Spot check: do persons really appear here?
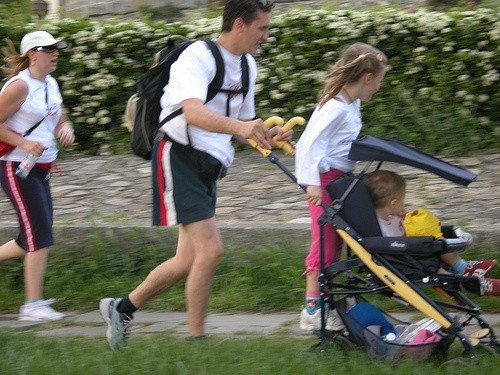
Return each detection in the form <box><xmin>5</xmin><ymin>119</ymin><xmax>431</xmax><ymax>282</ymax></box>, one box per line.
<box><xmin>295</xmin><ymin>43</ymin><xmax>388</xmax><ymax>332</ymax></box>
<box><xmin>99</xmin><ymin>0</ymin><xmax>293</xmax><ymax>352</ymax></box>
<box><xmin>362</xmin><ymin>170</ymin><xmax>500</xmax><ymax>296</ymax></box>
<box><xmin>0</xmin><ymin>30</ymin><xmax>77</xmax><ymax>322</ymax></box>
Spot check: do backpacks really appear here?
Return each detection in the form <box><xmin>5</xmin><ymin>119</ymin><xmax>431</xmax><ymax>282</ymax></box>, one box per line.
<box><xmin>127</xmin><ymin>34</ymin><xmax>251</xmax><ymax>158</ymax></box>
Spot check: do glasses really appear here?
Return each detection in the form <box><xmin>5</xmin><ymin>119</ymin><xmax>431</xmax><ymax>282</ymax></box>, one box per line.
<box><xmin>31</xmin><ymin>45</ymin><xmax>58</xmax><ymax>53</ymax></box>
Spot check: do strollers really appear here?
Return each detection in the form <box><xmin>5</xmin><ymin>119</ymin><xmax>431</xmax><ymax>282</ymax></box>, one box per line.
<box><xmin>246</xmin><ymin>115</ymin><xmax>500</xmax><ymax>367</ymax></box>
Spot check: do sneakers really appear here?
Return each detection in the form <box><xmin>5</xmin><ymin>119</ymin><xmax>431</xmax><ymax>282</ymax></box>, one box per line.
<box><xmin>17</xmin><ymin>298</ymin><xmax>65</xmax><ymax>322</ymax></box>
<box><xmin>476</xmin><ymin>277</ymin><xmax>500</xmax><ymax>297</ymax></box>
<box><xmin>462</xmin><ymin>258</ymin><xmax>497</xmax><ymax>278</ymax></box>
<box><xmin>299</xmin><ymin>307</ymin><xmax>345</xmax><ymax>331</ymax></box>
<box><xmin>99</xmin><ymin>298</ymin><xmax>136</xmax><ymax>351</ymax></box>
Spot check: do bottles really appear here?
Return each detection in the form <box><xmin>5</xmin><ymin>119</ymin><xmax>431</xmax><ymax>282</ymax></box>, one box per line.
<box><xmin>368</xmin><ymin>333</ymin><xmax>396</xmax><ymax>362</ymax></box>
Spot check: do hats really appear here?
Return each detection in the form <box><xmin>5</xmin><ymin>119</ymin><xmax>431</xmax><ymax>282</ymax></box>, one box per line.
<box><xmin>19</xmin><ymin>31</ymin><xmax>68</xmax><ymax>57</ymax></box>
